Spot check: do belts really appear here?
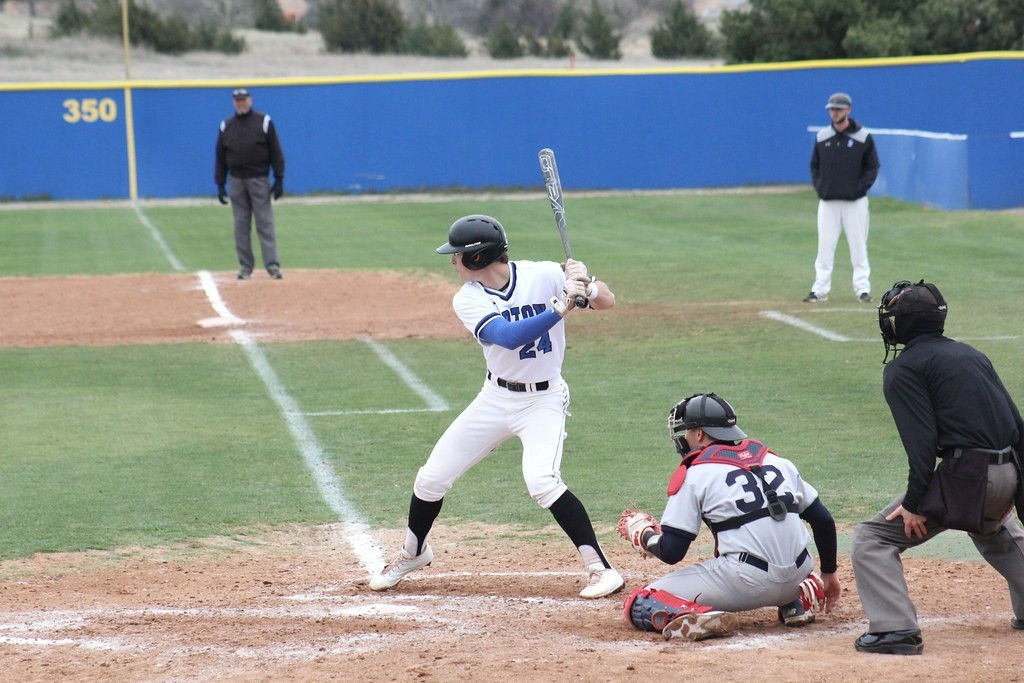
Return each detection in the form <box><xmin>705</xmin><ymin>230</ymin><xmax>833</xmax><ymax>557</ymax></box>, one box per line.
<box><xmin>948</xmin><ymin>447</ymin><xmax>1013</xmax><ymax>465</ymax></box>
<box><xmin>739</xmin><ymin>548</ymin><xmax>808</xmax><ymax>572</ymax></box>
<box><xmin>487</xmin><ymin>371</ymin><xmax>549</xmax><ymax>392</ymax></box>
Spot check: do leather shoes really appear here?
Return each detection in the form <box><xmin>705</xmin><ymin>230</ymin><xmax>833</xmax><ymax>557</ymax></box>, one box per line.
<box><xmin>854</xmin><ymin>630</ymin><xmax>924</xmax><ymax>654</ymax></box>
<box><xmin>1010</xmin><ymin>616</ymin><xmax>1023</xmax><ymax>629</ymax></box>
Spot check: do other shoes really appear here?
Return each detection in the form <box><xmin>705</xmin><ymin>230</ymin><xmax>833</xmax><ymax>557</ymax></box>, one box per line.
<box><xmin>269</xmin><ymin>270</ymin><xmax>282</xmax><ymax>277</ymax></box>
<box><xmin>238</xmin><ymin>271</ymin><xmax>249</xmax><ymax>278</ymax></box>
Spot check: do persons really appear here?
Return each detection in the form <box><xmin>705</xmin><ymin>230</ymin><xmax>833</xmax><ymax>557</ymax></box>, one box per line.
<box><xmin>852</xmin><ymin>281</ymin><xmax>1024</xmax><ymax>655</ymax></box>
<box><xmin>803</xmin><ymin>92</ymin><xmax>880</xmax><ymax>302</ymax></box>
<box><xmin>370</xmin><ymin>214</ymin><xmax>625</xmax><ymax>599</ymax></box>
<box><xmin>214</xmin><ymin>87</ymin><xmax>285</xmax><ymax>280</ymax></box>
<box><xmin>617</xmin><ymin>392</ymin><xmax>841</xmax><ymax>642</ymax></box>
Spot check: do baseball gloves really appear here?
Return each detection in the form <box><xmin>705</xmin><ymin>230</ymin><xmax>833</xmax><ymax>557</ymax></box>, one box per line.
<box><xmin>615</xmin><ymin>509</ymin><xmax>661</xmax><ymax>560</ymax></box>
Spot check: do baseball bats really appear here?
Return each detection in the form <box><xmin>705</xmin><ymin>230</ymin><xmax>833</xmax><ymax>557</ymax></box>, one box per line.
<box><xmin>537</xmin><ymin>146</ymin><xmax>588</xmax><ymax>309</ymax></box>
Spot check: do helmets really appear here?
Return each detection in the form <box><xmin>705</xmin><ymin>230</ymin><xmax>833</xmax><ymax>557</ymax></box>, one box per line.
<box><xmin>437</xmin><ymin>214</ymin><xmax>509</xmax><ymax>270</ymax></box>
<box><xmin>682</xmin><ymin>395</ymin><xmax>747</xmax><ymax>441</ymax></box>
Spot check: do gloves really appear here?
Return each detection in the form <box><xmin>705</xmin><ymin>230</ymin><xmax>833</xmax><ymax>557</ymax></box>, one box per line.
<box><xmin>269</xmin><ymin>178</ymin><xmax>282</xmax><ymax>199</ymax></box>
<box><xmin>218</xmin><ymin>183</ymin><xmax>228</xmax><ymax>204</ymax></box>
<box><xmin>550</xmin><ymin>277</ymin><xmax>592</xmax><ymax>316</ymax></box>
<box><xmin>560</xmin><ymin>257</ymin><xmax>596</xmax><ymax>295</ymax></box>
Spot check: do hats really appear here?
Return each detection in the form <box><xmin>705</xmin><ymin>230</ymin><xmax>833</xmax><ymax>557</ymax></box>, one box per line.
<box><xmin>232</xmin><ymin>88</ymin><xmax>249</xmax><ymax>98</ymax></box>
<box><xmin>885</xmin><ymin>285</ymin><xmax>947</xmax><ymax>323</ymax></box>
<box><xmin>825</xmin><ymin>93</ymin><xmax>851</xmax><ymax>109</ymax></box>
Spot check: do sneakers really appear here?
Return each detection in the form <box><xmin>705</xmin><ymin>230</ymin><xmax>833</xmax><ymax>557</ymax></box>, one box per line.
<box><xmin>803</xmin><ymin>292</ymin><xmax>828</xmax><ymax>303</ymax></box>
<box><xmin>778</xmin><ymin>600</ymin><xmax>816</xmax><ymax>626</ymax></box>
<box><xmin>579</xmin><ymin>567</ymin><xmax>625</xmax><ymax>599</ymax></box>
<box><xmin>367</xmin><ymin>543</ymin><xmax>433</xmax><ymax>590</ymax></box>
<box><xmin>859</xmin><ymin>293</ymin><xmax>872</xmax><ymax>302</ymax></box>
<box><xmin>662</xmin><ymin>611</ymin><xmax>737</xmax><ymax>642</ymax></box>
